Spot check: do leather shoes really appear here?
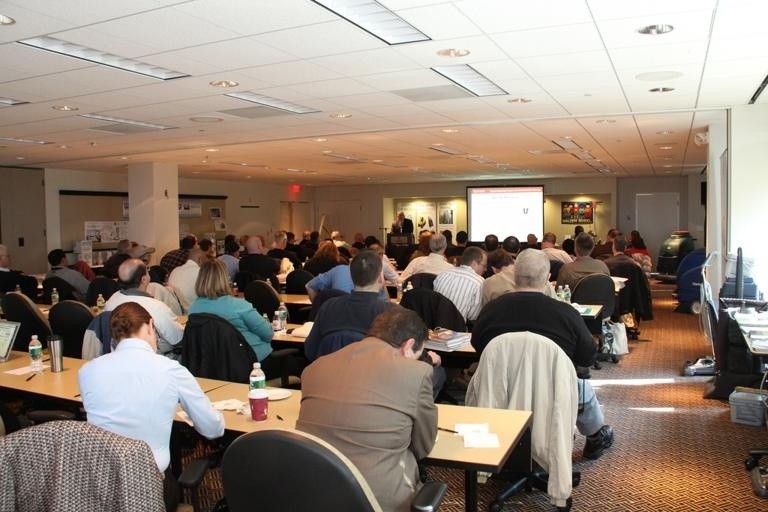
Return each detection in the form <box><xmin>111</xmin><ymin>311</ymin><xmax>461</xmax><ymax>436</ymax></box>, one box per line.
<box><xmin>584</xmin><ymin>425</ymin><xmax>615</xmax><ymax>459</ymax></box>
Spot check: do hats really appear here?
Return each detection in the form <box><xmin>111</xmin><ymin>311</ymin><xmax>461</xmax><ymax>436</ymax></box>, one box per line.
<box><xmin>128</xmin><ymin>246</ymin><xmax>155</xmax><ymax>259</ymax></box>
<box><xmin>224</xmin><ymin>240</ymin><xmax>239</xmax><ymax>252</ymax></box>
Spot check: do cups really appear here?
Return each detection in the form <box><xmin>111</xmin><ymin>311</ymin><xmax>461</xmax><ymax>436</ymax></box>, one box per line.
<box><xmin>47</xmin><ymin>336</ymin><xmax>64</xmax><ymax>373</ymax></box>
<box><xmin>248</xmin><ymin>389</ymin><xmax>269</xmax><ymax>421</ymax></box>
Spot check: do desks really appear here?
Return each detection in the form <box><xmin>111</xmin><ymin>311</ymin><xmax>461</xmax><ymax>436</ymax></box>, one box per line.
<box><xmin>0</xmin><ymin>258</ymin><xmax>629</xmax><ymax>512</ymax></box>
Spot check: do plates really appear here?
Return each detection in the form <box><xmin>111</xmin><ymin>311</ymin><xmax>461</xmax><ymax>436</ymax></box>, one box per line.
<box><xmin>268</xmin><ymin>389</ymin><xmax>293</xmax><ymax>401</ymax></box>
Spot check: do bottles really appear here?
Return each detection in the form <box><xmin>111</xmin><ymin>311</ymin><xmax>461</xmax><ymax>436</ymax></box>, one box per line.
<box><xmin>15</xmin><ymin>284</ymin><xmax>21</xmax><ymax>294</ymax></box>
<box><xmin>51</xmin><ymin>287</ymin><xmax>59</xmax><ymax>305</ymax></box>
<box><xmin>556</xmin><ymin>284</ymin><xmax>571</xmax><ymax>304</ymax></box>
<box><xmin>96</xmin><ymin>293</ymin><xmax>105</xmax><ymax>312</ymax></box>
<box><xmin>262</xmin><ymin>301</ymin><xmax>288</xmax><ymax>332</ymax></box>
<box><xmin>232</xmin><ymin>282</ymin><xmax>238</xmax><ymax>297</ymax></box>
<box><xmin>397</xmin><ymin>279</ymin><xmax>413</xmax><ymax>302</ymax></box>
<box><xmin>249</xmin><ymin>361</ymin><xmax>266</xmax><ymax>390</ymax></box>
<box><xmin>28</xmin><ymin>334</ymin><xmax>42</xmax><ymax>372</ymax></box>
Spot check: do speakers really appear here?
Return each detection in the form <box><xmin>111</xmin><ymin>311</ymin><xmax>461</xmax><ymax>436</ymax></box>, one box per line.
<box><xmin>701</xmin><ymin>181</ymin><xmax>706</xmax><ymax>204</ymax></box>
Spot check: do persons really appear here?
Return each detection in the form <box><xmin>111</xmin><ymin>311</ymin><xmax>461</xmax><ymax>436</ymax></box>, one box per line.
<box><xmin>78</xmin><ymin>302</ymin><xmax>225</xmax><ymax>512</ymax></box>
<box><xmin>295</xmin><ymin>308</ymin><xmax>439</xmax><ymax>512</ymax></box>
<box><xmin>391</xmin><ymin>212</ymin><xmax>413</xmax><ymax>235</ymax></box>
<box><xmin>1</xmin><ymin>225</ymin><xmax>650</xmax><ymax>459</ymax></box>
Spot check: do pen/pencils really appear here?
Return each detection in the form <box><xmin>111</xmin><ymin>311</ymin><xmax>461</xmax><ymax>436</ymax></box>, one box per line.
<box><xmin>437</xmin><ymin>426</ymin><xmax>458</xmax><ymax>433</ymax></box>
<box><xmin>26</xmin><ymin>374</ymin><xmax>36</xmax><ymax>381</ymax></box>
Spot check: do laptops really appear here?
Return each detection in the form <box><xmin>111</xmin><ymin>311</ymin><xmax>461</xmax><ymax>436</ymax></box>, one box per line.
<box><xmin>0</xmin><ymin>320</ymin><xmax>21</xmax><ymax>361</ymax></box>
